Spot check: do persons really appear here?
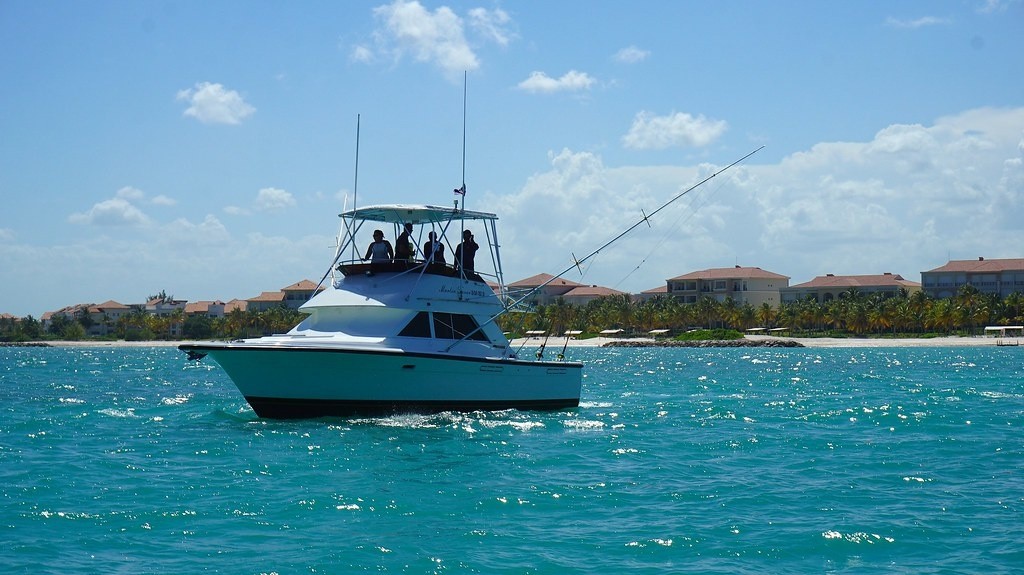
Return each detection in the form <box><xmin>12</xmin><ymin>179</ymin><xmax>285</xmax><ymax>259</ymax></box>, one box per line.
<box><xmin>365</xmin><ymin>230</ymin><xmax>394</xmax><ymax>263</ymax></box>
<box><xmin>454</xmin><ymin>230</ymin><xmax>479</xmax><ymax>274</ymax></box>
<box><xmin>424</xmin><ymin>231</ymin><xmax>446</xmax><ymax>266</ymax></box>
<box><xmin>394</xmin><ymin>223</ymin><xmax>415</xmax><ymax>263</ymax></box>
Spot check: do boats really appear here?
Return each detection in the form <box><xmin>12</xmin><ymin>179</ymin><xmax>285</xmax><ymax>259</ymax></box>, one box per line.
<box><xmin>177</xmin><ymin>69</ymin><xmax>768</xmax><ymax>420</ymax></box>
